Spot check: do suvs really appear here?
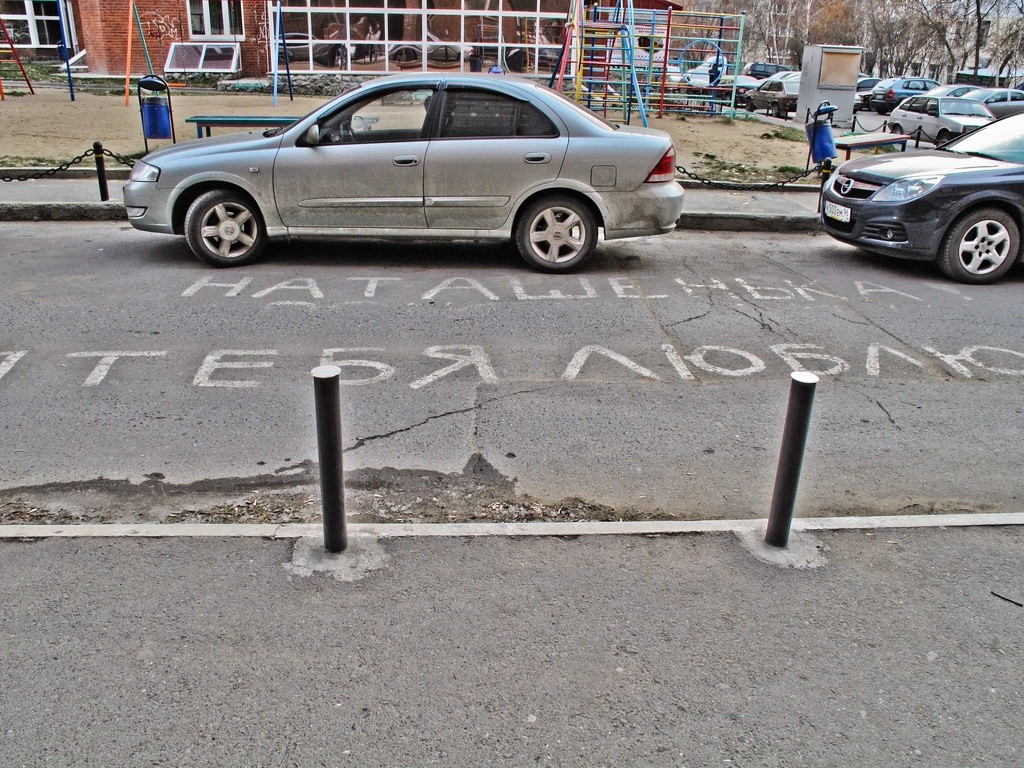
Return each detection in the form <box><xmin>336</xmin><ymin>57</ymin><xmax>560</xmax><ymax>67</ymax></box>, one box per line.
<box><xmin>870</xmin><ymin>75</ymin><xmax>943</xmax><ymax>115</ymax></box>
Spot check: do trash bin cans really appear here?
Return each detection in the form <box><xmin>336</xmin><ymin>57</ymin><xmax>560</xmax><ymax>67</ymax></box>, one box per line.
<box><xmin>804</xmin><ymin>106</ymin><xmax>839</xmax><ymax>164</ymax></box>
<box><xmin>138</xmin><ymin>78</ymin><xmax>172</xmax><ymax>140</ymax></box>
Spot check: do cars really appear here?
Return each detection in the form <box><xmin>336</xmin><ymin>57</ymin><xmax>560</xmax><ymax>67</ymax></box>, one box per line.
<box><xmin>714</xmin><ymin>62</ymin><xmax>883</xmax><ymax>111</ymax></box>
<box><xmin>923</xmin><ymin>84</ymin><xmax>982</xmax><ymax>98</ymax></box>
<box><xmin>821</xmin><ymin>110</ymin><xmax>1024</xmax><ymax>284</ymax></box>
<box><xmin>275</xmin><ymin>31</ymin><xmax>349</xmax><ymax>66</ymax></box>
<box><xmin>958</xmin><ymin>85</ymin><xmax>1024</xmax><ymax>118</ymax></box>
<box><xmin>387</xmin><ymin>39</ymin><xmax>474</xmax><ymax>64</ymax></box>
<box><xmin>745</xmin><ymin>80</ymin><xmax>801</xmax><ymax>117</ymax></box>
<box><xmin>887</xmin><ymin>95</ymin><xmax>998</xmax><ymax>147</ymax></box>
<box><xmin>123</xmin><ymin>68</ymin><xmax>688</xmax><ymax>273</ymax></box>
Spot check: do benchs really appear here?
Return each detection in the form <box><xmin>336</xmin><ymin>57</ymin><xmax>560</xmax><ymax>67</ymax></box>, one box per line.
<box><xmin>185</xmin><ymin>114</ymin><xmax>378</xmax><ymax>139</ymax></box>
<box><xmin>835</xmin><ymin>132</ymin><xmax>912</xmax><ymax>161</ymax></box>
